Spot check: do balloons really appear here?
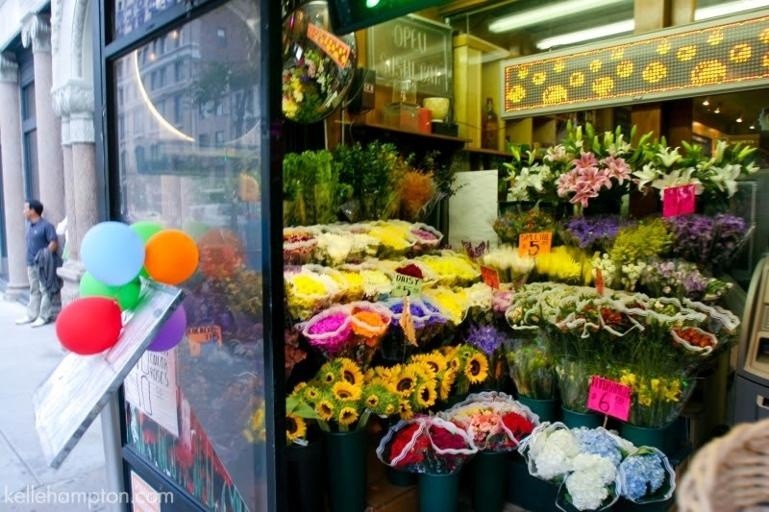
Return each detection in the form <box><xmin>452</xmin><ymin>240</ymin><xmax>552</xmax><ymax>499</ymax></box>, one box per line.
<box><xmin>147</xmin><ymin>300</ymin><xmax>187</xmax><ymax>354</ymax></box>
<box><xmin>54</xmin><ymin>297</ymin><xmax>121</xmax><ymax>354</ymax></box>
<box><xmin>144</xmin><ymin>227</ymin><xmax>199</xmax><ymax>289</ymax></box>
<box><xmin>77</xmin><ymin>270</ymin><xmax>141</xmax><ymax>313</ymax></box>
<box><xmin>78</xmin><ymin>220</ymin><xmax>144</xmax><ymax>287</ymax></box>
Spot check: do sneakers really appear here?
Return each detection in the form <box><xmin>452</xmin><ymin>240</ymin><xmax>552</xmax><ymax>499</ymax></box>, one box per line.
<box><xmin>15</xmin><ymin>315</ymin><xmax>47</xmax><ymax>327</ymax></box>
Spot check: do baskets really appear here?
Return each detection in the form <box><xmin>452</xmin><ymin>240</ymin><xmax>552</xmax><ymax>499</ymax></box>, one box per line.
<box><xmin>674</xmin><ymin>417</ymin><xmax>769</xmax><ymax>512</ymax></box>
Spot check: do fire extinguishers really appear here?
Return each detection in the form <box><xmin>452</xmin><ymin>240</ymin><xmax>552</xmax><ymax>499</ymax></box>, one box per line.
<box><xmin>482</xmin><ymin>98</ymin><xmax>498</xmax><ymax>150</ymax></box>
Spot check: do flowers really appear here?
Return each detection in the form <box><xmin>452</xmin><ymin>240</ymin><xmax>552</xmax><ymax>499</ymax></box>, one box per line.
<box><xmin>280</xmin><ymin>1</ymin><xmax>769</xmax><ymax>512</ymax></box>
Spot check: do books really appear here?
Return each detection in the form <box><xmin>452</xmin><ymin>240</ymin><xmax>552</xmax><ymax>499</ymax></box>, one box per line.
<box><xmin>129</xmin><ymin>218</ymin><xmax>165</xmax><ymax>245</ymax></box>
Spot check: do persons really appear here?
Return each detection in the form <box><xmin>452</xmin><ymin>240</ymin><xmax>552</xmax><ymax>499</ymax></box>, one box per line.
<box><xmin>15</xmin><ymin>201</ymin><xmax>61</xmax><ymax>328</ymax></box>
